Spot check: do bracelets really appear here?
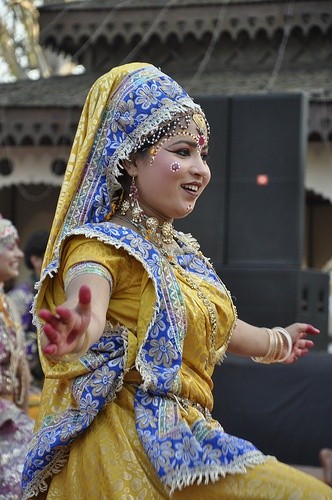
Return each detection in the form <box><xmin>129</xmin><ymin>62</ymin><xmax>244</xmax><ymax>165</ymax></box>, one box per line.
<box><xmin>249</xmin><ymin>325</ymin><xmax>294</xmax><ymax>365</ymax></box>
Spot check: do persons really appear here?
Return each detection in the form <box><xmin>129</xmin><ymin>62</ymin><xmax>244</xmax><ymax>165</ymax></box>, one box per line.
<box><xmin>33</xmin><ymin>64</ymin><xmax>330</xmax><ymax>498</ymax></box>
<box><xmin>1</xmin><ymin>215</ymin><xmax>37</xmax><ymax>419</ymax></box>
